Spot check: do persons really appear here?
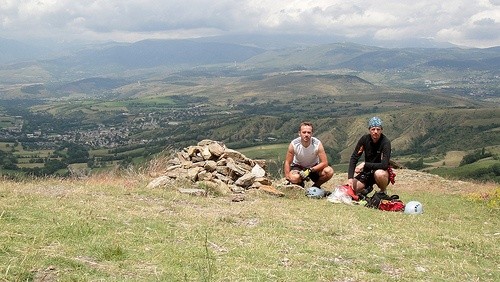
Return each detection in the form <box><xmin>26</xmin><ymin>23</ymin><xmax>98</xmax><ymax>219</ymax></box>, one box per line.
<box><xmin>284</xmin><ymin>122</ymin><xmax>334</xmax><ymax>190</ymax></box>
<box><xmin>346</xmin><ymin>117</ymin><xmax>392</xmax><ymax>200</ymax></box>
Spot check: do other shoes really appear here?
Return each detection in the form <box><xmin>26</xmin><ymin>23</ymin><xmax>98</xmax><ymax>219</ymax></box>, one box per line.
<box><xmin>358</xmin><ymin>188</ymin><xmax>371</xmax><ymax>200</ymax></box>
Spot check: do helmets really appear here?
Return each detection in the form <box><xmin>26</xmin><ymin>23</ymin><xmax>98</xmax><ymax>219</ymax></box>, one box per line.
<box><xmin>404</xmin><ymin>201</ymin><xmax>423</xmax><ymax>214</ymax></box>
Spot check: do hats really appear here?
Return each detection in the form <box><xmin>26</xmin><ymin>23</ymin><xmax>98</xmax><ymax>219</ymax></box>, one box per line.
<box><xmin>368</xmin><ymin>117</ymin><xmax>382</xmax><ymax>129</ymax></box>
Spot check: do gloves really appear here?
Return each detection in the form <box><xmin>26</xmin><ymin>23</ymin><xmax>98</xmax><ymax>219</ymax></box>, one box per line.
<box><xmin>300</xmin><ymin>168</ymin><xmax>311</xmax><ymax>179</ymax></box>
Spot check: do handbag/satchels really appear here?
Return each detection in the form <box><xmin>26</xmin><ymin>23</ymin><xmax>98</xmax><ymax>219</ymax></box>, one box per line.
<box><xmin>378</xmin><ymin>199</ymin><xmax>405</xmax><ymax>212</ymax></box>
<box><xmin>365</xmin><ymin>192</ymin><xmax>399</xmax><ymax>209</ymax></box>
